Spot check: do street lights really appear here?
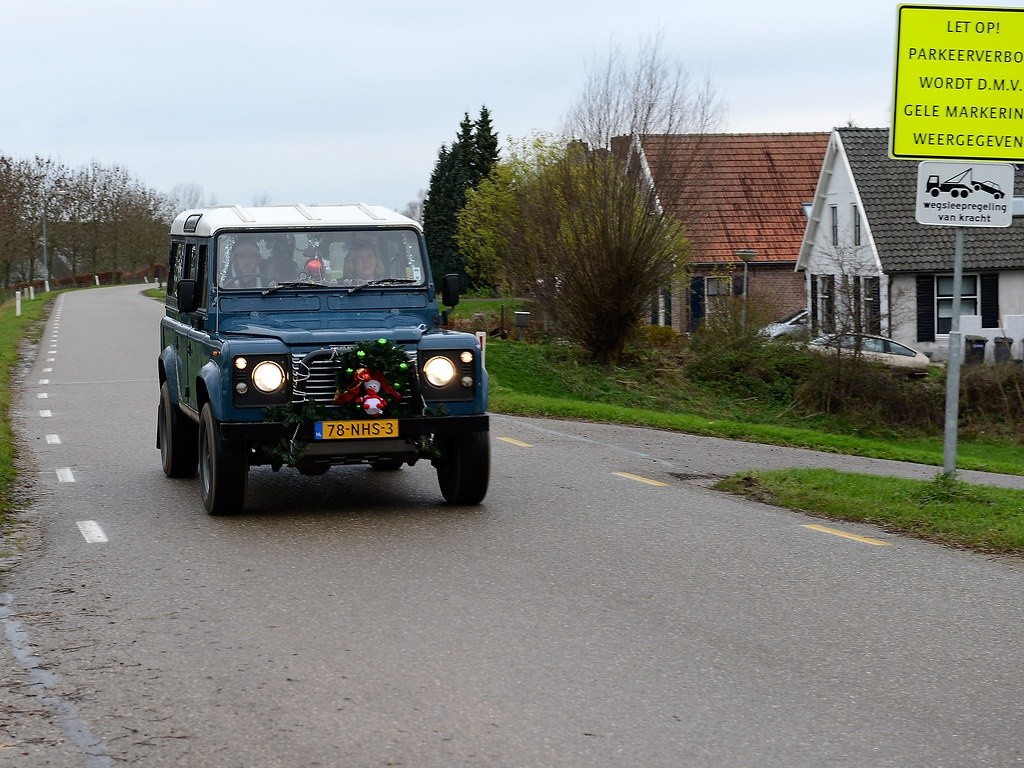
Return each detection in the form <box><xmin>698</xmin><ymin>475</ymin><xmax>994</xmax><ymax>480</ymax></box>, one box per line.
<box><xmin>800</xmin><ymin>202</ymin><xmax>819</xmax><ymax>341</ymax></box>
<box><xmin>734</xmin><ymin>248</ymin><xmax>759</xmax><ymax>334</ymax></box>
<box><xmin>43</xmin><ymin>190</ymin><xmax>70</xmax><ymax>292</ymax></box>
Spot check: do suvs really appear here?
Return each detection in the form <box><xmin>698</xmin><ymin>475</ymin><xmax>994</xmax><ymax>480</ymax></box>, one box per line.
<box><xmin>155</xmin><ymin>203</ymin><xmax>491</xmax><ymax>516</ymax></box>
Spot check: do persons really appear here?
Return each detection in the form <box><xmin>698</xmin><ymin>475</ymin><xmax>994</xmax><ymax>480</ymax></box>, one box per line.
<box><xmin>337</xmin><ymin>237</ymin><xmax>390</xmax><ymax>284</ymax></box>
<box><xmin>221</xmin><ymin>237</ymin><xmax>279</xmax><ymax>288</ymax></box>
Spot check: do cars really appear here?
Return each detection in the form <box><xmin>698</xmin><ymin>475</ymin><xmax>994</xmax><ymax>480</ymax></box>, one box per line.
<box><xmin>758</xmin><ymin>308</ymin><xmax>809</xmax><ymax>341</ymax></box>
<box><xmin>806</xmin><ymin>332</ymin><xmax>930</xmax><ymax>370</ymax></box>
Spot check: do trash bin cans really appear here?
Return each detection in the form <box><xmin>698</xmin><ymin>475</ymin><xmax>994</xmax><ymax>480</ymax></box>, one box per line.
<box><xmin>964</xmin><ymin>335</ymin><xmax>988</xmax><ymax>366</ymax></box>
<box><xmin>994</xmin><ymin>337</ymin><xmax>1013</xmax><ymax>364</ymax></box>
